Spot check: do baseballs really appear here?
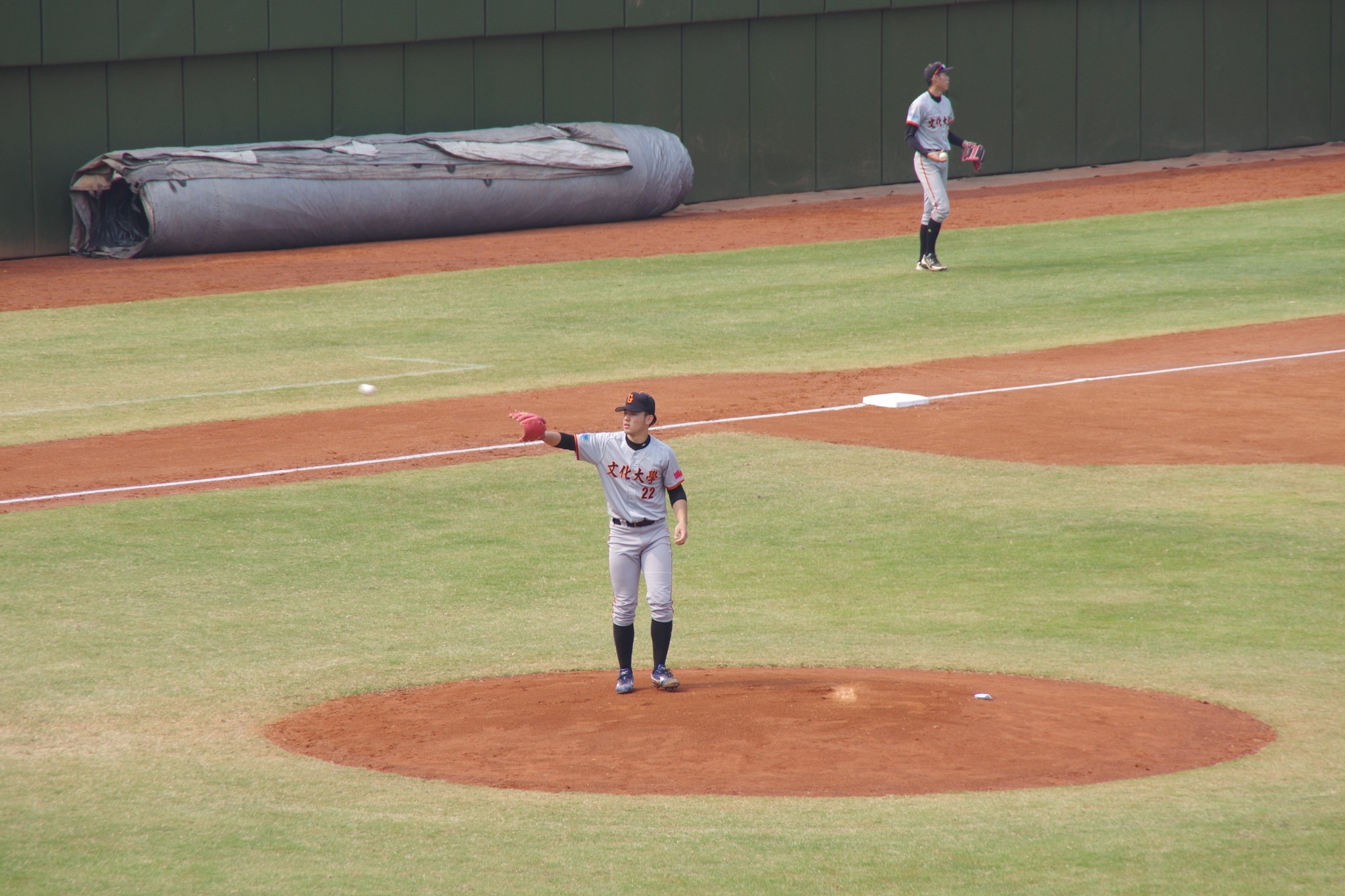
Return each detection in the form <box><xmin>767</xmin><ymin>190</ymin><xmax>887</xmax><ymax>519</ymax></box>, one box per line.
<box><xmin>939</xmin><ymin>152</ymin><xmax>948</xmax><ymax>160</ymax></box>
<box><xmin>357</xmin><ymin>383</ymin><xmax>377</xmax><ymax>396</ymax></box>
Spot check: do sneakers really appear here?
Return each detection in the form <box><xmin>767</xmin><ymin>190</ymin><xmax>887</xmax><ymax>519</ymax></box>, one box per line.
<box><xmin>616</xmin><ymin>668</ymin><xmax>634</xmax><ymax>693</ymax></box>
<box><xmin>916</xmin><ymin>261</ymin><xmax>947</xmax><ymax>270</ymax></box>
<box><xmin>921</xmin><ymin>253</ymin><xmax>943</xmax><ymax>271</ymax></box>
<box><xmin>651</xmin><ymin>664</ymin><xmax>679</xmax><ymax>688</ymax></box>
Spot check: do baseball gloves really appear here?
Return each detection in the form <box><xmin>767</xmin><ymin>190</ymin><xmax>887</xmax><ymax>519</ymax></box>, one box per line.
<box><xmin>508</xmin><ymin>412</ymin><xmax>547</xmax><ymax>444</ymax></box>
<box><xmin>961</xmin><ymin>142</ymin><xmax>985</xmax><ymax>172</ymax></box>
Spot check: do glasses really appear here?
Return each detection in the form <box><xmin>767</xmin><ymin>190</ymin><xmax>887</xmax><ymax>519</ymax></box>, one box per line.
<box><xmin>933</xmin><ymin>64</ymin><xmax>945</xmax><ymax>75</ymax></box>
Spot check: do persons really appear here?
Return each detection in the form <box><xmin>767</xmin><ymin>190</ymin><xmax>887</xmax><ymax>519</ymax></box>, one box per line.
<box><xmin>509</xmin><ymin>392</ymin><xmax>689</xmax><ymax>694</ymax></box>
<box><xmin>905</xmin><ymin>61</ymin><xmax>985</xmax><ymax>272</ymax></box>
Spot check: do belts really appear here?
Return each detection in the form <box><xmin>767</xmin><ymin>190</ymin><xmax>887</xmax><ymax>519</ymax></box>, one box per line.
<box><xmin>927</xmin><ymin>149</ymin><xmax>948</xmax><ymax>152</ymax></box>
<box><xmin>613</xmin><ymin>517</ymin><xmax>655</xmax><ymax>528</ymax></box>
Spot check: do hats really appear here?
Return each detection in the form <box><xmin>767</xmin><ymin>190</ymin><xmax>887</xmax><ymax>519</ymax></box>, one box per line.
<box><xmin>615</xmin><ymin>391</ymin><xmax>655</xmax><ymax>413</ymax></box>
<box><xmin>924</xmin><ymin>61</ymin><xmax>953</xmax><ymax>80</ymax></box>
